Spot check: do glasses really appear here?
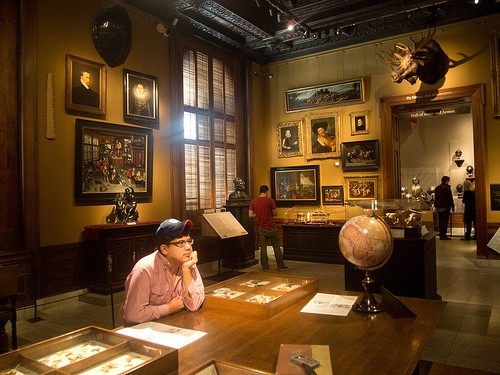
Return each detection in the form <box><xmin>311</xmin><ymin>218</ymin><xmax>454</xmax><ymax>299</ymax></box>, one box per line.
<box><xmin>162</xmin><ymin>239</ymin><xmax>195</xmax><ymax>248</ymax></box>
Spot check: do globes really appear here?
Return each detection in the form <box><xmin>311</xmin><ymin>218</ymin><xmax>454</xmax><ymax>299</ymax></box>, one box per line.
<box><xmin>338</xmin><ymin>211</ymin><xmax>394</xmax><ymax>313</ymax></box>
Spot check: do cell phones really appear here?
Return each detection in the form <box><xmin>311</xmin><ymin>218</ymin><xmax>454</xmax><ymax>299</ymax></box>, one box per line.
<box><xmin>291</xmin><ymin>354</ymin><xmax>320</xmax><ymax>368</ymax></box>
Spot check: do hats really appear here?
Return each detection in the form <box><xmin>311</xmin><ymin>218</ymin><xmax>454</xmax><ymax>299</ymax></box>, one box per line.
<box><xmin>156</xmin><ymin>218</ymin><xmax>193</xmax><ymax>241</ymax></box>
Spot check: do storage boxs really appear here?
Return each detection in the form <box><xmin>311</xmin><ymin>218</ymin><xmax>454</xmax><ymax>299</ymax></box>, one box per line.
<box><xmin>0</xmin><ymin>325</ymin><xmax>178</xmax><ymax>375</ymax></box>
<box><xmin>202</xmin><ymin>272</ymin><xmax>319</xmax><ymax>319</ymax></box>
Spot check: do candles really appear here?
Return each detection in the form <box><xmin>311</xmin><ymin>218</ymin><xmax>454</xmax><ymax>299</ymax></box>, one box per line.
<box><xmin>371</xmin><ymin>199</ymin><xmax>378</xmax><ymax>210</ymax></box>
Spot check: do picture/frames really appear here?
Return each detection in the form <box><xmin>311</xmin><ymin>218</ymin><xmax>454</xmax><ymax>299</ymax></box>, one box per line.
<box><xmin>283</xmin><ymin>77</ymin><xmax>366</xmax><ymax>114</ymax></box>
<box><xmin>340</xmin><ymin>139</ymin><xmax>381</xmax><ymax>171</ymax></box>
<box><xmin>270</xmin><ymin>164</ymin><xmax>320</xmax><ymax>206</ymax></box>
<box><xmin>321</xmin><ymin>185</ymin><xmax>344</xmax><ymax>205</ymax></box>
<box><xmin>305</xmin><ymin>111</ymin><xmax>341</xmax><ymax>161</ymax></box>
<box><xmin>74</xmin><ymin>119</ymin><xmax>153</xmax><ymax>203</ymax></box>
<box><xmin>276</xmin><ymin>120</ymin><xmax>304</xmax><ymax>158</ymax></box>
<box><xmin>123</xmin><ymin>68</ymin><xmax>160</xmax><ymax>130</ymax></box>
<box><xmin>65</xmin><ymin>54</ymin><xmax>107</xmax><ymax>116</ymax></box>
<box><xmin>350</xmin><ymin>110</ymin><xmax>370</xmax><ymax>135</ymax></box>
<box><xmin>343</xmin><ymin>175</ymin><xmax>379</xmax><ymax>201</ymax></box>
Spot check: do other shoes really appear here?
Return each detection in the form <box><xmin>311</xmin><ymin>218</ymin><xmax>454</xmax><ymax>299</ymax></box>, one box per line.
<box><xmin>471</xmin><ymin>235</ymin><xmax>477</xmax><ymax>240</ymax></box>
<box><xmin>440</xmin><ymin>236</ymin><xmax>451</xmax><ymax>240</ymax></box>
<box><xmin>278</xmin><ymin>266</ymin><xmax>289</xmax><ymax>271</ymax></box>
<box><xmin>461</xmin><ymin>237</ymin><xmax>470</xmax><ymax>241</ymax></box>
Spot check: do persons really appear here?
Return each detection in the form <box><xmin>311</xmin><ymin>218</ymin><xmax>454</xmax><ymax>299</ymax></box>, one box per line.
<box><xmin>229</xmin><ymin>176</ymin><xmax>248</xmax><ymax>198</ymax></box>
<box><xmin>460</xmin><ymin>180</ymin><xmax>475</xmax><ymax>240</ymax></box>
<box><xmin>249</xmin><ymin>185</ymin><xmax>288</xmax><ymax>271</ymax></box>
<box><xmin>122</xmin><ymin>187</ymin><xmax>139</xmax><ymax>221</ymax></box>
<box><xmin>118</xmin><ymin>218</ymin><xmax>205</xmax><ymax>324</ymax></box>
<box><xmin>435</xmin><ymin>176</ymin><xmax>454</xmax><ymax>240</ymax></box>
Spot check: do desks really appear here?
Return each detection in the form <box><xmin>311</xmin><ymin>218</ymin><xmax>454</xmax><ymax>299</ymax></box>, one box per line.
<box><xmin>223</xmin><ymin>204</ymin><xmax>259</xmax><ymax>269</ymax></box>
<box><xmin>111</xmin><ymin>287</ymin><xmax>445</xmax><ymax>375</ymax></box>
<box><xmin>413</xmin><ymin>210</ymin><xmax>454</xmax><ymax>237</ymax></box>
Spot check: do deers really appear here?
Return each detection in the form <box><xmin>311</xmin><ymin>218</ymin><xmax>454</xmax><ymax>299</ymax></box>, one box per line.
<box><xmin>376</xmin><ymin>26</ymin><xmax>450</xmax><ymax>86</ymax></box>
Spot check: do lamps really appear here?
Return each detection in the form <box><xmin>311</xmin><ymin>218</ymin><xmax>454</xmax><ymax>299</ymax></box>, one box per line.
<box><xmin>286</xmin><ymin>15</ymin><xmax>298</xmax><ymax>31</ymax></box>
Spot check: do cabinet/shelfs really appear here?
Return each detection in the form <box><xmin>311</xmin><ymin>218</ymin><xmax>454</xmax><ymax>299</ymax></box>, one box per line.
<box><xmin>83</xmin><ymin>220</ymin><xmax>159</xmax><ymax>296</ymax></box>
<box><xmin>280</xmin><ymin>221</ymin><xmax>346</xmax><ymax>264</ymax></box>
<box><xmin>343</xmin><ymin>230</ymin><xmax>442</xmax><ymax>299</ymax></box>
